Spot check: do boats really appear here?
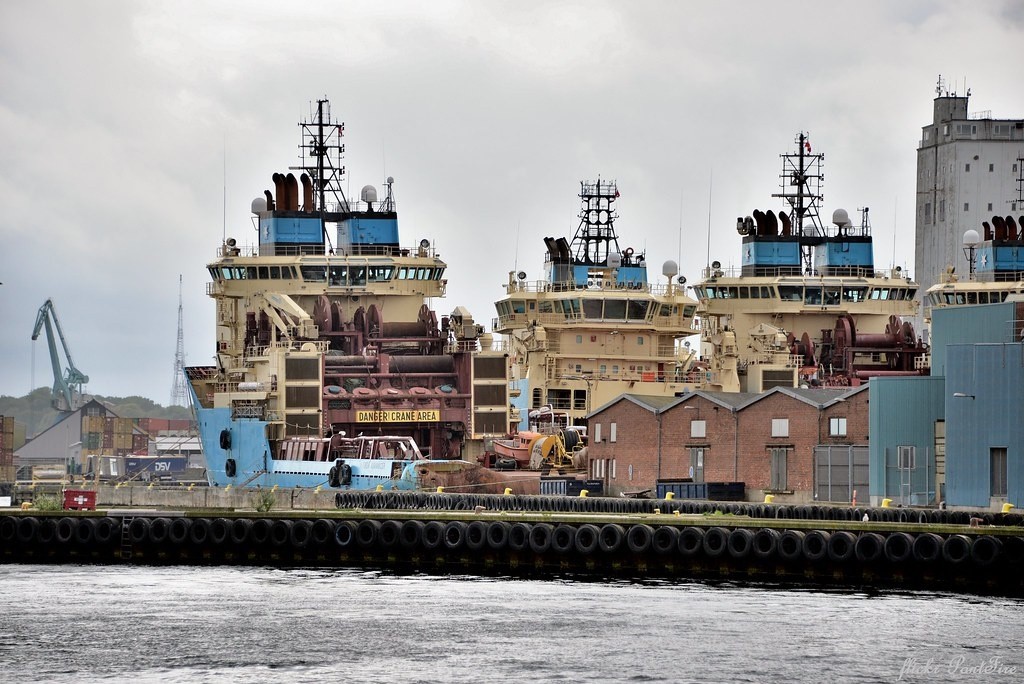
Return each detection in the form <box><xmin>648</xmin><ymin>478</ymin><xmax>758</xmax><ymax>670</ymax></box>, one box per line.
<box><xmin>493</xmin><ymin>431</ymin><xmax>541</xmax><ymax>469</ymax></box>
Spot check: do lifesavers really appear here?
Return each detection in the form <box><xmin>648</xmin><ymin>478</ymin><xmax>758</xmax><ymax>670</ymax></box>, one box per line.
<box><xmin>338</xmin><ymin>465</ymin><xmax>353</xmax><ymax>484</ymax></box>
<box><xmin>1002</xmin><ymin>536</ymin><xmax>1024</xmax><ymax>570</ymax></box>
<box><xmin>3</xmin><ymin>516</ymin><xmax>803</xmax><ymax>559</ymax></box>
<box><xmin>941</xmin><ymin>534</ymin><xmax>971</xmax><ymax>567</ymax></box>
<box><xmin>828</xmin><ymin>531</ymin><xmax>856</xmax><ymax>560</ymax></box>
<box><xmin>801</xmin><ymin>531</ymin><xmax>829</xmax><ymax>559</ymax></box>
<box><xmin>220</xmin><ymin>430</ymin><xmax>231</xmax><ymax>450</ymax></box>
<box><xmin>225</xmin><ymin>458</ymin><xmax>238</xmax><ymax>477</ymax></box>
<box><xmin>856</xmin><ymin>533</ymin><xmax>884</xmax><ymax>564</ymax></box>
<box><xmin>913</xmin><ymin>533</ymin><xmax>943</xmax><ymax>564</ymax></box>
<box><xmin>884</xmin><ymin>532</ymin><xmax>913</xmax><ymax>562</ymax></box>
<box><xmin>328</xmin><ymin>467</ymin><xmax>339</xmax><ymax>487</ymax></box>
<box><xmin>970</xmin><ymin>535</ymin><xmax>1002</xmax><ymax>568</ymax></box>
